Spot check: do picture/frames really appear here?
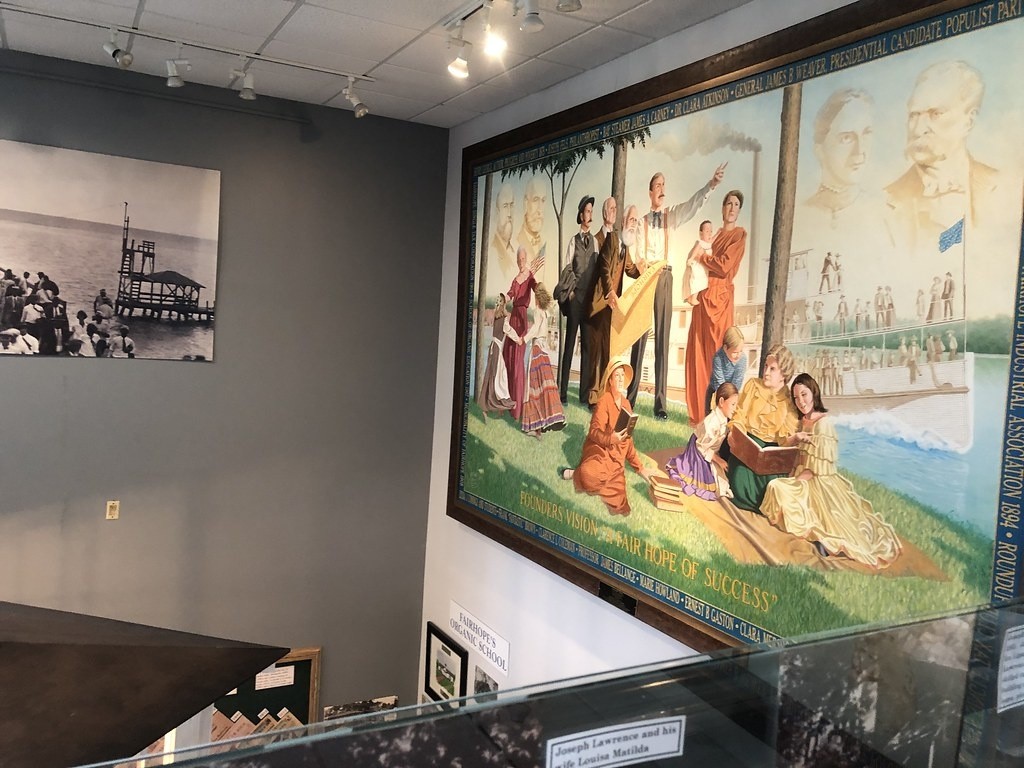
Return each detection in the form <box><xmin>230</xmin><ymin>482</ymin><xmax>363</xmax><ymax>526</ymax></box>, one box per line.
<box><xmin>423</xmin><ymin>621</ymin><xmax>469</xmax><ymax>712</ymax></box>
<box><xmin>445</xmin><ymin>0</ymin><xmax>1024</xmax><ymax>658</ymax></box>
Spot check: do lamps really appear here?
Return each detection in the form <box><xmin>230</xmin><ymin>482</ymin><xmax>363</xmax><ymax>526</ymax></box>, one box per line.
<box><xmin>520</xmin><ymin>0</ymin><xmax>544</xmax><ymax>34</ymax></box>
<box><xmin>555</xmin><ymin>0</ymin><xmax>582</xmax><ymax>14</ymax></box>
<box><xmin>164</xmin><ymin>59</ymin><xmax>192</xmax><ymax>88</ymax></box>
<box><xmin>102</xmin><ymin>41</ymin><xmax>134</xmax><ymax>69</ymax></box>
<box><xmin>347</xmin><ymin>91</ymin><xmax>369</xmax><ymax>118</ymax></box>
<box><xmin>446</xmin><ymin>40</ymin><xmax>472</xmax><ymax>79</ymax></box>
<box><xmin>230</xmin><ymin>70</ymin><xmax>257</xmax><ymax>100</ymax></box>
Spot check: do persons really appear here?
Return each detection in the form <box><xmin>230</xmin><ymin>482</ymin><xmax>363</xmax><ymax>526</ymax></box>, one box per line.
<box><xmin>1</xmin><ymin>268</ymin><xmax>207</xmax><ymax>362</ymax></box>
